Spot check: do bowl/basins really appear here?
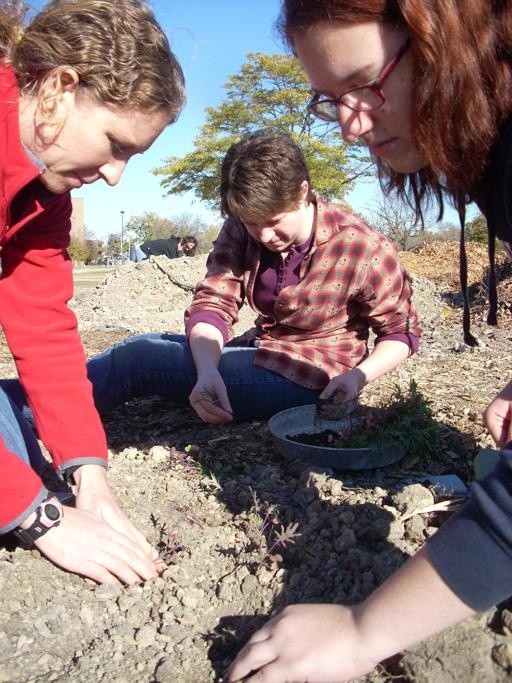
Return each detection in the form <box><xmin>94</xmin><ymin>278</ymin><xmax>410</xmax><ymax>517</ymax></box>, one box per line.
<box><xmin>269</xmin><ymin>402</ymin><xmax>411</xmax><ymax>471</ymax></box>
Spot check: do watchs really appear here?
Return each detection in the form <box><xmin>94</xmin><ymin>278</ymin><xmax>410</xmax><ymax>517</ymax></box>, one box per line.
<box><xmin>12</xmin><ymin>495</ymin><xmax>64</xmax><ymax>547</ymax></box>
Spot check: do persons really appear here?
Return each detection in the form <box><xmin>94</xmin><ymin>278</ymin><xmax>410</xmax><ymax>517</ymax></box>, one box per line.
<box><xmin>136</xmin><ymin>236</ymin><xmax>197</xmax><ymax>264</ymax></box>
<box><xmin>223</xmin><ymin>1</ymin><xmax>512</xmax><ymax>683</ymax></box>
<box><xmin>3</xmin><ymin>129</ymin><xmax>421</xmax><ymax>439</ymax></box>
<box><xmin>1</xmin><ymin>0</ymin><xmax>185</xmax><ymax>587</ymax></box>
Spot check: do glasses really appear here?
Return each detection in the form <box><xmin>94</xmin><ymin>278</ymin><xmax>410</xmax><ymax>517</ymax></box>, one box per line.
<box><xmin>306</xmin><ymin>34</ymin><xmax>414</xmax><ymax>122</ymax></box>
